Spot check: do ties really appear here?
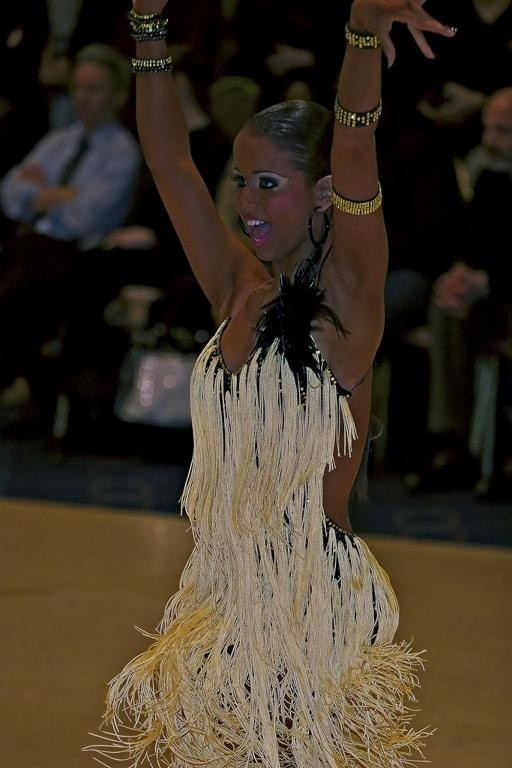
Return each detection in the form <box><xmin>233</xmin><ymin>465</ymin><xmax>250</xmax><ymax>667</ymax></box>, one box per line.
<box><xmin>58</xmin><ymin>137</ymin><xmax>90</xmax><ymax>186</ymax></box>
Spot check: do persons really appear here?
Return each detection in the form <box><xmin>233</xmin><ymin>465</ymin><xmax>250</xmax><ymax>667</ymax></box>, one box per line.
<box><xmin>86</xmin><ymin>1</ymin><xmax>456</xmax><ymax>768</ymax></box>
